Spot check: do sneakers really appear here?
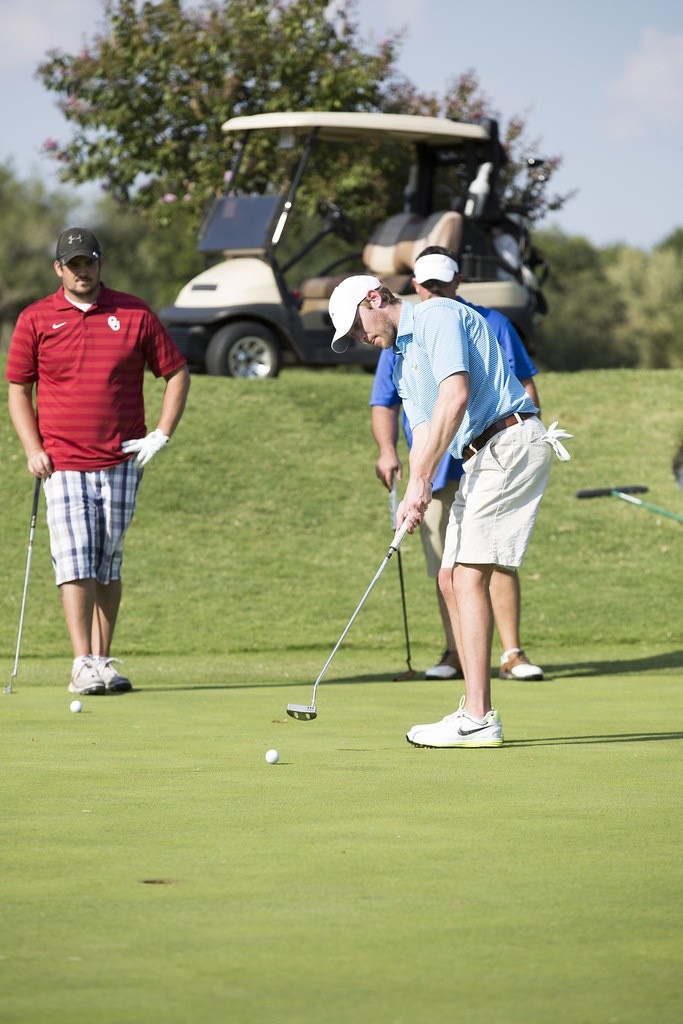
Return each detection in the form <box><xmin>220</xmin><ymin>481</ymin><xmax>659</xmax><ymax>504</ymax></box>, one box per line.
<box><xmin>424</xmin><ymin>651</ymin><xmax>462</xmax><ymax>680</ymax></box>
<box><xmin>499</xmin><ymin>650</ymin><xmax>543</xmax><ymax>680</ymax></box>
<box><xmin>95</xmin><ymin>657</ymin><xmax>133</xmax><ymax>692</ymax></box>
<box><xmin>406</xmin><ymin>695</ymin><xmax>503</xmax><ymax>747</ymax></box>
<box><xmin>68</xmin><ymin>656</ymin><xmax>107</xmax><ymax>695</ymax></box>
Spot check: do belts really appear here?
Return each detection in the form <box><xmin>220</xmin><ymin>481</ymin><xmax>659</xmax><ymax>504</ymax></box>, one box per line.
<box><xmin>462</xmin><ymin>413</ymin><xmax>535</xmax><ymax>460</ymax></box>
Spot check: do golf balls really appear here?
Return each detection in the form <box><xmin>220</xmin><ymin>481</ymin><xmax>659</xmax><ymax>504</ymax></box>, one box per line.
<box><xmin>265</xmin><ymin>750</ymin><xmax>279</xmax><ymax>764</ymax></box>
<box><xmin>70</xmin><ymin>701</ymin><xmax>82</xmax><ymax>713</ymax></box>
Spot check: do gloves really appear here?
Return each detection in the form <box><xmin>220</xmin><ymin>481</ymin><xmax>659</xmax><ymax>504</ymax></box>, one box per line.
<box><xmin>539</xmin><ymin>422</ymin><xmax>575</xmax><ymax>462</ymax></box>
<box><xmin>121</xmin><ymin>429</ymin><xmax>169</xmax><ymax>468</ymax></box>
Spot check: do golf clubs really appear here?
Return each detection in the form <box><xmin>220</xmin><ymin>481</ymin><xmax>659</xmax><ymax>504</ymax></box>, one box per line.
<box><xmin>390</xmin><ymin>477</ymin><xmax>416</xmax><ymax>682</ymax></box>
<box><xmin>3</xmin><ymin>478</ymin><xmax>43</xmax><ymax>694</ymax></box>
<box><xmin>287</xmin><ymin>483</ymin><xmax>435</xmax><ymax>721</ymax></box>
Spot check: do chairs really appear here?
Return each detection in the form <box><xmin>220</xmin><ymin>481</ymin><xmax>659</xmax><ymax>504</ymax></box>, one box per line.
<box><xmin>301</xmin><ymin>210</ymin><xmax>461</xmax><ymax>312</ymax></box>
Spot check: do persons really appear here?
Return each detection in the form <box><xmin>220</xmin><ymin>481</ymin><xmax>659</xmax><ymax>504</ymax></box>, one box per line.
<box><xmin>369</xmin><ymin>245</ymin><xmax>544</xmax><ymax>680</ymax></box>
<box><xmin>7</xmin><ymin>228</ymin><xmax>191</xmax><ymax>696</ymax></box>
<box><xmin>329</xmin><ymin>275</ymin><xmax>554</xmax><ymax>748</ymax></box>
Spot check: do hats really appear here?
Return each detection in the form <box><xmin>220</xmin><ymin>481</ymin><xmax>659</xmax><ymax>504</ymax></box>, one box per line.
<box><xmin>328</xmin><ymin>275</ymin><xmax>382</xmax><ymax>353</ymax></box>
<box><xmin>57</xmin><ymin>227</ymin><xmax>101</xmax><ymax>266</ymax></box>
<box><xmin>413</xmin><ymin>253</ymin><xmax>460</xmax><ymax>284</ymax></box>
